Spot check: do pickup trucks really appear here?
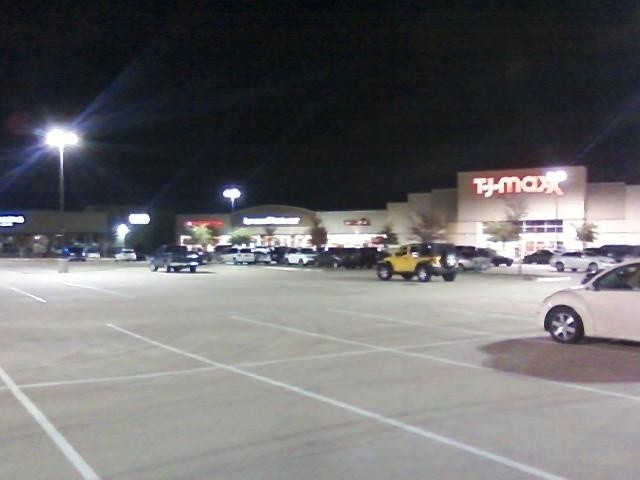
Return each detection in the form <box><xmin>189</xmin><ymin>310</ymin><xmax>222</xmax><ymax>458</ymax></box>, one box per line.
<box><xmin>149</xmin><ymin>244</ymin><xmax>199</xmax><ymax>273</ymax></box>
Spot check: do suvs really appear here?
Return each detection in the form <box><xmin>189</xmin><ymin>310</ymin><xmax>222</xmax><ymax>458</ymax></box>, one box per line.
<box><xmin>376</xmin><ymin>242</ymin><xmax>456</xmax><ymax>282</ymax></box>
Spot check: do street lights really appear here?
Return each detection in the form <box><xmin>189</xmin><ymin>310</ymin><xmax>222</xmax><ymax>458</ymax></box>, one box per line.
<box><xmin>545</xmin><ymin>169</ymin><xmax>569</xmax><ymax>251</ymax></box>
<box><xmin>45</xmin><ymin>128</ymin><xmax>79</xmax><ymax>210</ymax></box>
<box><xmin>222</xmin><ymin>187</ymin><xmax>242</xmax><ymax>213</ymax></box>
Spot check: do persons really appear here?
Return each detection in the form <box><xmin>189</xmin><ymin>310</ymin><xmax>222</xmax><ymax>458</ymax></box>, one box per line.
<box><xmin>581</xmin><ymin>262</ymin><xmax>603</xmax><ymax>289</ymax></box>
<box><xmin>553</xmin><ymin>241</ymin><xmax>566</xmax><ymax>253</ymax></box>
<box><xmin>610</xmin><ymin>271</ymin><xmax>633</xmax><ymax>291</ymax></box>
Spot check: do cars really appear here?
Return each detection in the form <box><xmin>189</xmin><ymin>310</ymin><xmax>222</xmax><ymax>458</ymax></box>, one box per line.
<box><xmin>537</xmin><ymin>261</ymin><xmax>640</xmax><ymax>344</ymax></box>
<box><xmin>254</xmin><ymin>248</ymin><xmax>272</xmax><ymax>264</ymax></box>
<box><xmin>189</xmin><ymin>248</ymin><xmax>213</xmax><ymax>265</ymax></box>
<box><xmin>272</xmin><ymin>246</ymin><xmax>378</xmax><ymax>270</ymax></box>
<box><xmin>0</xmin><ymin>240</ymin><xmax>138</xmax><ymax>261</ymax></box>
<box><xmin>456</xmin><ymin>245</ymin><xmax>640</xmax><ymax>273</ymax></box>
<box><xmin>219</xmin><ymin>247</ymin><xmax>256</xmax><ymax>264</ymax></box>
